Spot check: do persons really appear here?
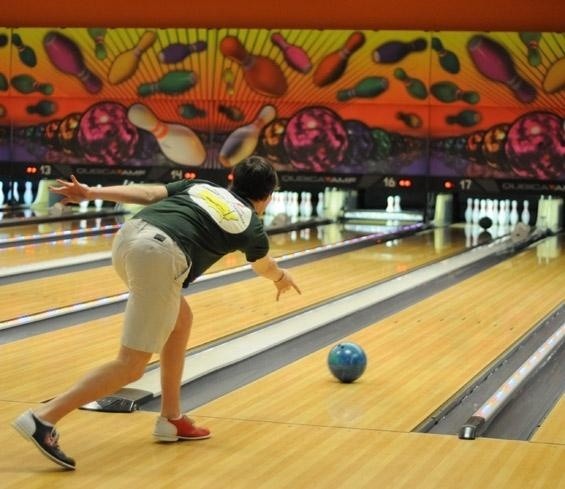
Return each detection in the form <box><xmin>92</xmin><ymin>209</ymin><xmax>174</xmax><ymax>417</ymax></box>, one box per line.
<box><xmin>10</xmin><ymin>154</ymin><xmax>302</xmax><ymax>471</ymax></box>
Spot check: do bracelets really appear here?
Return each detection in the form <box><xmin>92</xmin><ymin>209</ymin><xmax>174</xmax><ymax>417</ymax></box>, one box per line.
<box><xmin>274</xmin><ymin>270</ymin><xmax>284</xmax><ymax>283</ymax></box>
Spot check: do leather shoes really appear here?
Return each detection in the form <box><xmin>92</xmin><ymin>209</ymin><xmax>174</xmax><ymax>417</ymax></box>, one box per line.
<box><xmin>152</xmin><ymin>415</ymin><xmax>212</xmax><ymax>442</ymax></box>
<box><xmin>9</xmin><ymin>408</ymin><xmax>76</xmax><ymax>471</ymax></box>
